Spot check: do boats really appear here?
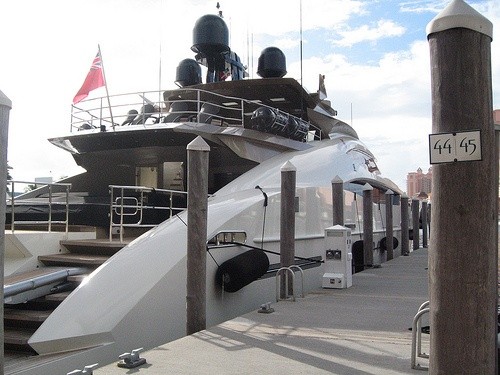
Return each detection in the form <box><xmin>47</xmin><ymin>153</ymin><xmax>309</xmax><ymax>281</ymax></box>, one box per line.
<box><xmin>1</xmin><ymin>0</ymin><xmax>417</xmax><ymax>375</ymax></box>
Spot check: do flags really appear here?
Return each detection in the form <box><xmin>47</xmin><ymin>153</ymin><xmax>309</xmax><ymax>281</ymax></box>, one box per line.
<box><xmin>73</xmin><ymin>49</ymin><xmax>106</xmax><ymax>105</ymax></box>
